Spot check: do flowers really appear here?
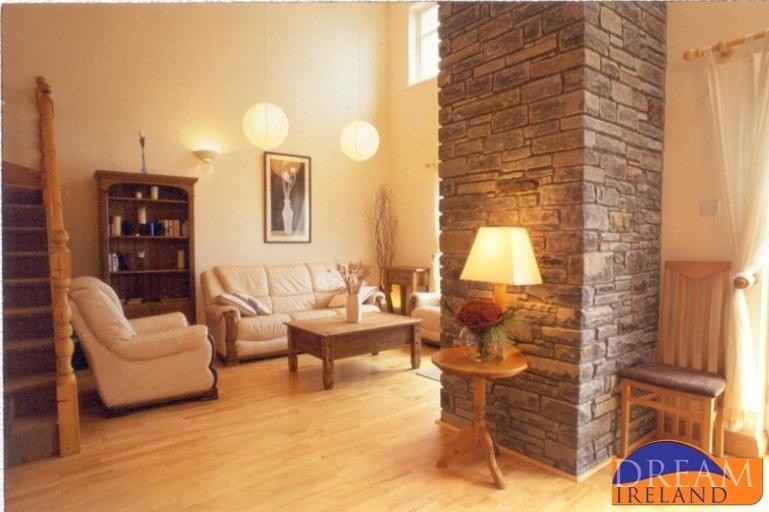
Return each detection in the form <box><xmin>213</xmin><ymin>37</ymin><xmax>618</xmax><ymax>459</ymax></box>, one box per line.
<box><xmin>451</xmin><ymin>300</ymin><xmax>504</xmax><ymax>326</ymax></box>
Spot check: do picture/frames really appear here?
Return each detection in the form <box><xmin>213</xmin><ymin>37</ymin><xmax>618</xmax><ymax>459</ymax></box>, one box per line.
<box><xmin>264</xmin><ymin>151</ymin><xmax>313</xmax><ymax>243</ymax></box>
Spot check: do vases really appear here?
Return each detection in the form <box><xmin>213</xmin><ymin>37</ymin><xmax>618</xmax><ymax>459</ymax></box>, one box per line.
<box><xmin>459</xmin><ymin>326</ymin><xmax>506</xmax><ymax>364</ymax></box>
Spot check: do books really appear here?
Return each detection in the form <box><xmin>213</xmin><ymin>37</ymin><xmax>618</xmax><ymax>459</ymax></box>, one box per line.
<box><xmin>159</xmin><ymin>217</ymin><xmax>182</xmax><ymax>236</ymax></box>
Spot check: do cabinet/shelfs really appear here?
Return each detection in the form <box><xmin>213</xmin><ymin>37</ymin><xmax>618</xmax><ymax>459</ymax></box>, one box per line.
<box><xmin>93</xmin><ymin>169</ymin><xmax>198</xmax><ymax>325</ymax></box>
<box><xmin>2</xmin><ymin>162</ymin><xmax>81</xmax><ymax>470</ymax></box>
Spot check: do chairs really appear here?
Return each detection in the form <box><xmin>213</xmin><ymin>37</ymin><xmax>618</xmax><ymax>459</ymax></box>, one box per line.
<box><xmin>69</xmin><ymin>276</ymin><xmax>219</xmax><ymax>419</ymax></box>
<box><xmin>619</xmin><ymin>260</ymin><xmax>733</xmax><ymax>459</ymax></box>
<box><xmin>409</xmin><ymin>253</ymin><xmax>440</xmax><ymax>349</ymax></box>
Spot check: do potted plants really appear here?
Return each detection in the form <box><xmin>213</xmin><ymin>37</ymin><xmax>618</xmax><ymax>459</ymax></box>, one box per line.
<box><xmin>334</xmin><ymin>263</ymin><xmax>372</xmax><ymax>323</ymax></box>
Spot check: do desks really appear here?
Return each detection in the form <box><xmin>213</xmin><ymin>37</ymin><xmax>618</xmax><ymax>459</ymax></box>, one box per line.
<box><xmin>431</xmin><ymin>347</ymin><xmax>529</xmax><ymax>489</ymax></box>
<box><xmin>388</xmin><ymin>267</ymin><xmax>429</xmax><ymax>314</ymax></box>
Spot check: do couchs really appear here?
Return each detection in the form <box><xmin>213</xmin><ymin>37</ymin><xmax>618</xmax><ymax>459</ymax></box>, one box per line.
<box><xmin>200</xmin><ymin>262</ymin><xmax>386</xmax><ymax>367</ymax></box>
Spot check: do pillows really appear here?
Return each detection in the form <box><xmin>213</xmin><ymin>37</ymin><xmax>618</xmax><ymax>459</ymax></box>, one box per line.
<box><xmin>233</xmin><ymin>292</ymin><xmax>269</xmax><ymax>315</ymax></box>
<box><xmin>328</xmin><ymin>286</ymin><xmax>375</xmax><ymax>308</ymax></box>
<box><xmin>217</xmin><ymin>293</ymin><xmax>257</xmax><ymax>316</ymax></box>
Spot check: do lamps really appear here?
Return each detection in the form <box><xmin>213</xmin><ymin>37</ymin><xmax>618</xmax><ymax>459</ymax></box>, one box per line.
<box><xmin>193</xmin><ymin>149</ymin><xmax>219</xmax><ymax>163</ymax></box>
<box><xmin>460</xmin><ymin>227</ymin><xmax>543</xmax><ymax>311</ymax></box>
<box><xmin>242</xmin><ymin>4</ymin><xmax>290</xmax><ymax>151</ymax></box>
<box><xmin>340</xmin><ymin>3</ymin><xmax>380</xmax><ymax>161</ymax></box>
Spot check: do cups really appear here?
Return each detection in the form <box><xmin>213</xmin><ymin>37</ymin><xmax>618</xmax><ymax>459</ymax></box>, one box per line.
<box><xmin>108</xmin><ymin>253</ymin><xmax>120</xmax><ymax>273</ymax></box>
<box><xmin>146</xmin><ymin>221</ymin><xmax>161</xmax><ymax>236</ymax></box>
